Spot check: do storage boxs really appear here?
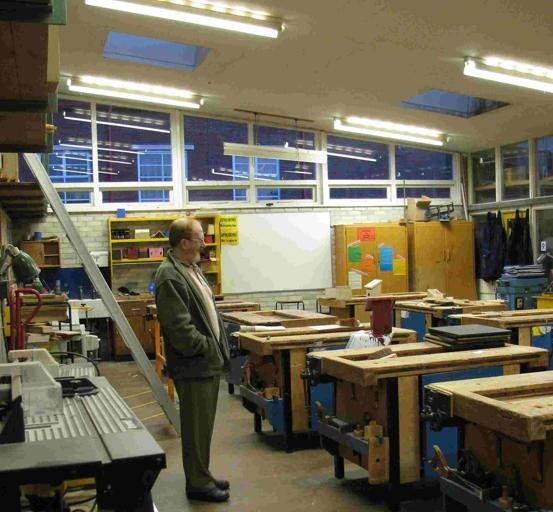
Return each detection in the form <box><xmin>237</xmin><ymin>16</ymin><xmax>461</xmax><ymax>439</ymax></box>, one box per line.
<box><xmin>404</xmin><ymin>195</ymin><xmax>432</xmax><ymax>221</ymax></box>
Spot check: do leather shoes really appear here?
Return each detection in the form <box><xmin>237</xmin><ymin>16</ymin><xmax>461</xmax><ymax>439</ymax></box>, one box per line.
<box><xmin>186</xmin><ymin>486</ymin><xmax>229</xmax><ymax>502</ymax></box>
<box><xmin>214</xmin><ymin>479</ymin><xmax>229</xmax><ymax>490</ymax></box>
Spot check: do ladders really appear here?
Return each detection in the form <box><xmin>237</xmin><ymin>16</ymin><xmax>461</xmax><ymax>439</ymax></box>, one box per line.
<box><xmin>23</xmin><ymin>153</ymin><xmax>181</xmax><ymax>437</ymax></box>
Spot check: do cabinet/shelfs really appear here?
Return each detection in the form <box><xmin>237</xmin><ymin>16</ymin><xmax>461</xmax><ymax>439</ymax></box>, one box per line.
<box><xmin>107</xmin><ymin>214</ymin><xmax>223</xmax><ymax>356</ymax></box>
<box><xmin>18</xmin><ymin>240</ymin><xmax>62</xmax><ymax>268</ymax></box>
<box><xmin>331</xmin><ymin>218</ymin><xmax>478</xmax><ymax>302</ymax></box>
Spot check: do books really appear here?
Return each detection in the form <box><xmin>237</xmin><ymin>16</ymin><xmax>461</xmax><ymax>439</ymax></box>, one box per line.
<box><xmin>500</xmin><ymin>263</ymin><xmax>545</xmax><ymax>277</ymax></box>
<box><xmin>422</xmin><ymin>323</ymin><xmax>513</xmax><ymax>350</ymax></box>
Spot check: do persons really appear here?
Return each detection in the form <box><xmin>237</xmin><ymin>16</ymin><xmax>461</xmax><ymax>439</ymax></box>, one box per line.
<box><xmin>152</xmin><ymin>215</ymin><xmax>236</xmax><ymax>502</ymax></box>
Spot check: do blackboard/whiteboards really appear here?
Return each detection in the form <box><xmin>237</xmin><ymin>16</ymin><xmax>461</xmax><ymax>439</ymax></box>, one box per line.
<box><xmin>220</xmin><ymin>211</ymin><xmax>334</xmax><ymax>295</ymax></box>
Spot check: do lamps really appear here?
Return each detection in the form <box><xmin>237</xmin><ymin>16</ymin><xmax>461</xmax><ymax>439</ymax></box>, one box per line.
<box><xmin>0</xmin><ymin>243</ymin><xmax>41</xmax><ymax>286</ymax></box>
<box><xmin>83</xmin><ymin>0</ymin><xmax>285</xmax><ymax>39</ymax></box>
<box><xmin>462</xmin><ymin>55</ymin><xmax>552</xmax><ymax>93</ymax></box>
<box><xmin>332</xmin><ymin>115</ymin><xmax>451</xmax><ymax>147</ymax></box>
<box><xmin>222</xmin><ymin>108</ymin><xmax>328</xmax><ymax>164</ymax></box>
<box><xmin>66</xmin><ymin>75</ymin><xmax>207</xmax><ymax>109</ymax></box>
